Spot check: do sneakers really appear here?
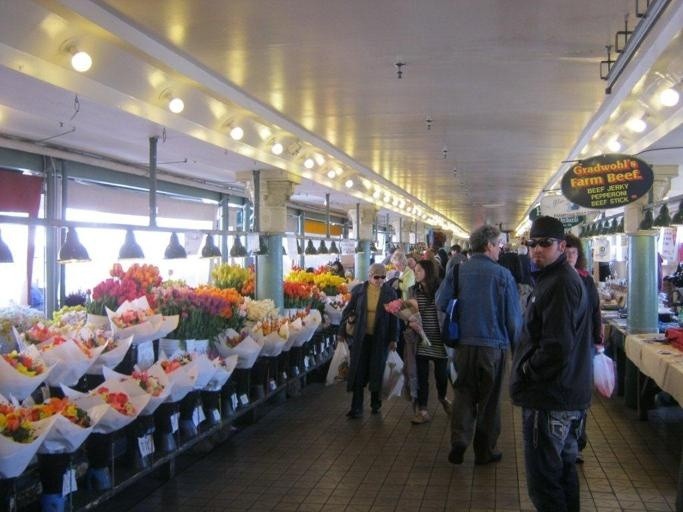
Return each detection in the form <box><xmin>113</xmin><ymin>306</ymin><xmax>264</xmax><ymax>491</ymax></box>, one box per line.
<box><xmin>438</xmin><ymin>396</ymin><xmax>453</xmax><ymax>415</ymax></box>
<box><xmin>449</xmin><ymin>443</ymin><xmax>466</xmax><ymax>465</ymax></box>
<box><xmin>475</xmin><ymin>450</ymin><xmax>502</xmax><ymax>465</ymax></box>
<box><xmin>410</xmin><ymin>412</ymin><xmax>431</xmax><ymax>424</ymax></box>
<box><xmin>575</xmin><ymin>451</ymin><xmax>584</xmax><ymax>462</ymax></box>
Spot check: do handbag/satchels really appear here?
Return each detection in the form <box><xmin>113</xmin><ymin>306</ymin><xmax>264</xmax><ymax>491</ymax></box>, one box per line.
<box><xmin>402</xmin><ymin>327</ymin><xmax>421</xmax><ymax>345</ymax></box>
<box><xmin>444</xmin><ymin>297</ymin><xmax>460</xmax><ymax>348</ymax></box>
<box><xmin>345</xmin><ymin>311</ymin><xmax>357</xmax><ymax>336</ymax></box>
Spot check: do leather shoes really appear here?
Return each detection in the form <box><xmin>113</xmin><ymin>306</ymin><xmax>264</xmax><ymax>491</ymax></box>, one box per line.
<box><xmin>371</xmin><ymin>408</ymin><xmax>381</xmax><ymax>413</ymax></box>
<box><xmin>346</xmin><ymin>408</ymin><xmax>363</xmax><ymax>416</ymax></box>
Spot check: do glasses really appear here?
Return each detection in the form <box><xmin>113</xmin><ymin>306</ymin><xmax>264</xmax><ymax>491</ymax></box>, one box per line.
<box><xmin>374</xmin><ymin>275</ymin><xmax>386</xmax><ymax>279</ymax></box>
<box><xmin>527</xmin><ymin>239</ymin><xmax>561</xmax><ymax>247</ymax></box>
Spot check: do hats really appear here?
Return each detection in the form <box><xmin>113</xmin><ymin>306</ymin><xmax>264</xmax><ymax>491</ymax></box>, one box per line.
<box><xmin>530</xmin><ymin>216</ymin><xmax>564</xmax><ymax>240</ymax></box>
<box><xmin>391</xmin><ymin>250</ymin><xmax>405</xmax><ymax>263</ymax></box>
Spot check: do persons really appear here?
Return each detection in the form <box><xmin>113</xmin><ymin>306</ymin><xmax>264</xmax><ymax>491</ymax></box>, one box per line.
<box><xmin>435</xmin><ymin>224</ymin><xmax>535</xmax><ymax>465</ymax></box>
<box><xmin>333</xmin><ymin>244</ymin><xmax>471</xmax><ymax>423</ymax></box>
<box><xmin>564</xmin><ymin>234</ymin><xmax>610</xmax><ymax>462</ymax></box>
<box><xmin>506</xmin><ymin>215</ymin><xmax>594</xmax><ymax>512</ymax></box>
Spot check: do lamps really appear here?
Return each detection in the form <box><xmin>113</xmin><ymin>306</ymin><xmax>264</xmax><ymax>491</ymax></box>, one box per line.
<box><xmin>578</xmin><ymin>163</ymin><xmax>683</xmax><ymax>239</ymax></box>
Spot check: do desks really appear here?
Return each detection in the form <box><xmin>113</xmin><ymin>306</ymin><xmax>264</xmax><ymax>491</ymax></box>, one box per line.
<box><xmin>625</xmin><ymin>330</ymin><xmax>683</xmax><ymax>422</ymax></box>
